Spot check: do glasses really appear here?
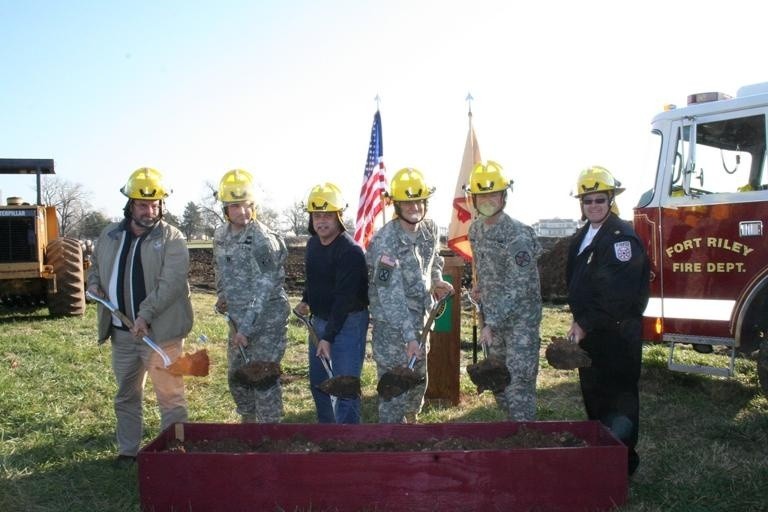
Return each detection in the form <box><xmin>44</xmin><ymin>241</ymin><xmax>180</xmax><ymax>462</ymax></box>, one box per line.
<box><xmin>581</xmin><ymin>196</ymin><xmax>611</xmax><ymax>204</ymax></box>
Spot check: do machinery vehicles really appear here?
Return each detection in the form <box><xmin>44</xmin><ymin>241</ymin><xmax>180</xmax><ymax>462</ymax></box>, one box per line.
<box><xmin>633</xmin><ymin>82</ymin><xmax>768</xmax><ymax>400</ymax></box>
<box><xmin>0</xmin><ymin>158</ymin><xmax>85</xmax><ymax>318</ymax></box>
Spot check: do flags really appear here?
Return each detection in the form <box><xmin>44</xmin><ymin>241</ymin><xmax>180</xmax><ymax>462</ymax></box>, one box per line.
<box><xmin>353</xmin><ymin>110</ymin><xmax>384</xmax><ymax>254</ymax></box>
<box><xmin>447</xmin><ymin>111</ymin><xmax>483</xmax><ymax>262</ymax></box>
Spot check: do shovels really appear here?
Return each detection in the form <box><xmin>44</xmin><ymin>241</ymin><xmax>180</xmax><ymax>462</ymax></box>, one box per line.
<box><xmin>215</xmin><ymin>303</ymin><xmax>279</xmax><ymax>391</ymax></box>
<box><xmin>466</xmin><ymin>292</ymin><xmax>512</xmax><ymax>393</ymax></box>
<box><xmin>86</xmin><ymin>289</ymin><xmax>206</xmax><ymax>375</ymax></box>
<box><xmin>546</xmin><ymin>315</ymin><xmax>592</xmax><ymax>370</ymax></box>
<box><xmin>377</xmin><ymin>286</ymin><xmax>453</xmax><ymax>398</ymax></box>
<box><xmin>293</xmin><ymin>306</ymin><xmax>362</xmax><ymax>399</ymax></box>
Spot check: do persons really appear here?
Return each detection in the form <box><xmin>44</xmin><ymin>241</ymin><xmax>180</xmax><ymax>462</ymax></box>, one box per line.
<box><xmin>293</xmin><ymin>183</ymin><xmax>369</xmax><ymax>423</ymax></box>
<box><xmin>211</xmin><ymin>169</ymin><xmax>291</xmax><ymax>423</ymax></box>
<box><xmin>567</xmin><ymin>166</ymin><xmax>651</xmax><ymax>475</ymax></box>
<box><xmin>365</xmin><ymin>168</ymin><xmax>453</xmax><ymax>424</ymax></box>
<box><xmin>464</xmin><ymin>160</ymin><xmax>545</xmax><ymax>422</ymax></box>
<box><xmin>84</xmin><ymin>168</ymin><xmax>193</xmax><ymax>469</ymax></box>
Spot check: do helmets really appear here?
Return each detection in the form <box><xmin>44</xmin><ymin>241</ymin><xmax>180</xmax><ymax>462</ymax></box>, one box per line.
<box><xmin>568</xmin><ymin>165</ymin><xmax>626</xmax><ymax>198</ymax></box>
<box><xmin>303</xmin><ymin>182</ymin><xmax>348</xmax><ymax>213</ymax></box>
<box><xmin>119</xmin><ymin>167</ymin><xmax>173</xmax><ymax>201</ymax></box>
<box><xmin>214</xmin><ymin>169</ymin><xmax>256</xmax><ymax>203</ymax></box>
<box><xmin>384</xmin><ymin>168</ymin><xmax>436</xmax><ymax>202</ymax></box>
<box><xmin>461</xmin><ymin>160</ymin><xmax>514</xmax><ymax>195</ymax></box>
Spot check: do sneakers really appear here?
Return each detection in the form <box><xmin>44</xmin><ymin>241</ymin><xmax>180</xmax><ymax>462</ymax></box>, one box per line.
<box><xmin>111</xmin><ymin>455</ymin><xmax>136</xmax><ymax>469</ymax></box>
